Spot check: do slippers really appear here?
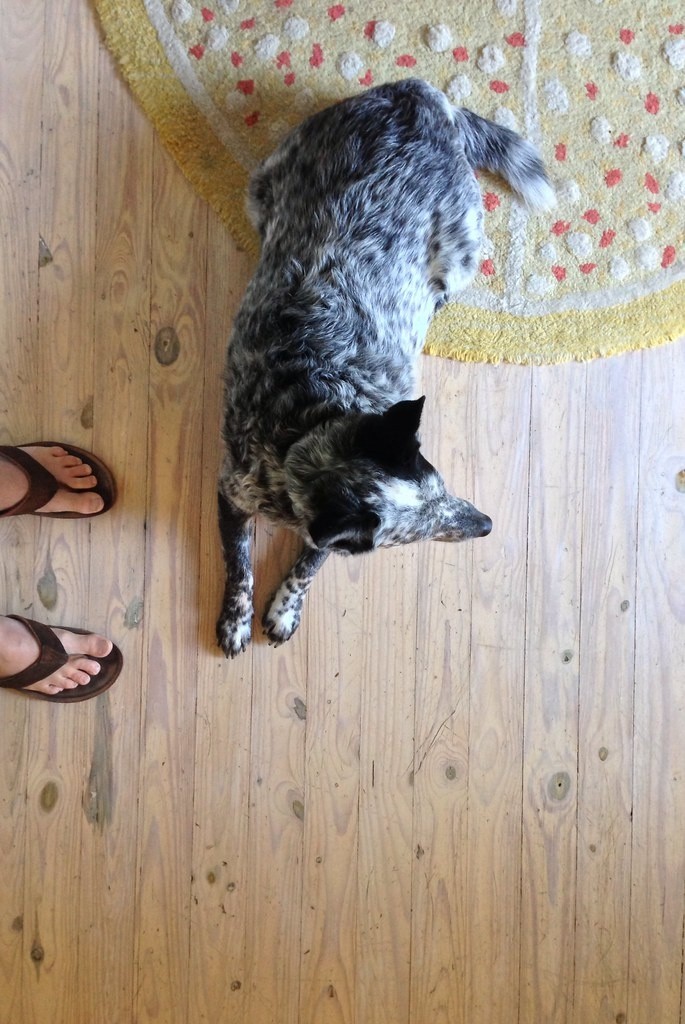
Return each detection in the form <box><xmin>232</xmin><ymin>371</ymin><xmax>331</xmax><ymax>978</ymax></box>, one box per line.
<box><xmin>0</xmin><ymin>614</ymin><xmax>124</xmax><ymax>703</ymax></box>
<box><xmin>0</xmin><ymin>441</ymin><xmax>117</xmax><ymax>519</ymax></box>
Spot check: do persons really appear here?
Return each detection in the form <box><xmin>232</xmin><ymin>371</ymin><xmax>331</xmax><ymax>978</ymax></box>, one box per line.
<box><xmin>0</xmin><ymin>441</ymin><xmax>123</xmax><ymax>704</ymax></box>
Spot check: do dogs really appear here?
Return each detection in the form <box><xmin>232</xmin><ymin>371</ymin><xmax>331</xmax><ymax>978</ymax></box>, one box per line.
<box><xmin>216</xmin><ymin>77</ymin><xmax>561</xmax><ymax>660</ymax></box>
